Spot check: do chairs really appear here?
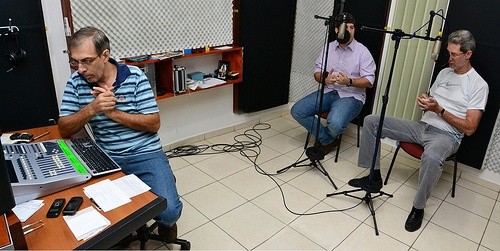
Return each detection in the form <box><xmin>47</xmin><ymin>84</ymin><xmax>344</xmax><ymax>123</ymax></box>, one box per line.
<box><xmin>304</xmin><ymin>111</ymin><xmax>360</xmax><ymax>162</ymax></box>
<box><xmin>384</xmin><ymin>142</ymin><xmax>457</xmax><ymax>198</ymax></box>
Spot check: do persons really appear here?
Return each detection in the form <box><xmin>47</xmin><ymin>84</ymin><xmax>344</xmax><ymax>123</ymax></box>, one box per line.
<box><xmin>291</xmin><ymin>14</ymin><xmax>376</xmax><ymax>159</ymax></box>
<box><xmin>348</xmin><ymin>30</ymin><xmax>489</xmax><ymax>232</ymax></box>
<box><xmin>58</xmin><ymin>26</ymin><xmax>183</xmax><ymax>248</ymax></box>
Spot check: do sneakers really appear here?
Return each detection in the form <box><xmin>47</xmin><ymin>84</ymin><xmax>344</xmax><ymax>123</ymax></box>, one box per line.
<box><xmin>405</xmin><ymin>206</ymin><xmax>424</xmax><ymax>232</ymax></box>
<box><xmin>315</xmin><ymin>137</ymin><xmax>339</xmax><ymax>156</ymax></box>
<box><xmin>348</xmin><ymin>174</ymin><xmax>382</xmax><ymax>189</ymax></box>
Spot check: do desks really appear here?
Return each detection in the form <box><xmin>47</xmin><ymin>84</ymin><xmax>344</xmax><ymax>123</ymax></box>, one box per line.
<box><xmin>0</xmin><ymin>126</ymin><xmax>167</xmax><ymax>251</ymax></box>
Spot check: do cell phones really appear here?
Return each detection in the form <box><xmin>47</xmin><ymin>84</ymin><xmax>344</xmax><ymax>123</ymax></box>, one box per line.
<box><xmin>62</xmin><ymin>197</ymin><xmax>83</xmax><ymax>216</ymax></box>
<box><xmin>46</xmin><ymin>198</ymin><xmax>65</xmax><ymax>218</ymax></box>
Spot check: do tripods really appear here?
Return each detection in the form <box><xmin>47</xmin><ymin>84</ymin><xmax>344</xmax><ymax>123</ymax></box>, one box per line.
<box><xmin>326</xmin><ymin>10</ymin><xmax>436</xmax><ymax>236</ymax></box>
<box><xmin>276</xmin><ymin>14</ymin><xmax>342</xmax><ymax>190</ymax></box>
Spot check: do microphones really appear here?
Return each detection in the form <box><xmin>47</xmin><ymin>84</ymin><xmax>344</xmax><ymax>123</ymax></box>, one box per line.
<box><xmin>431</xmin><ymin>31</ymin><xmax>443</xmax><ymax>61</ymax></box>
<box><xmin>338</xmin><ymin>14</ymin><xmax>347</xmax><ymax>40</ymax></box>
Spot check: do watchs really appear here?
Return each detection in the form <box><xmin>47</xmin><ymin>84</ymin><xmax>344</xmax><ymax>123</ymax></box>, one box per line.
<box><xmin>347</xmin><ymin>79</ymin><xmax>352</xmax><ymax>87</ymax></box>
<box><xmin>439</xmin><ymin>109</ymin><xmax>445</xmax><ymax>117</ymax></box>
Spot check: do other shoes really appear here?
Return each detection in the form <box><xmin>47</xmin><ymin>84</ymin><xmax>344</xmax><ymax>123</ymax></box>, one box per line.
<box><xmin>158</xmin><ymin>221</ymin><xmax>177</xmax><ymax>244</ymax></box>
<box><xmin>120</xmin><ymin>232</ymin><xmax>132</xmax><ymax>248</ymax></box>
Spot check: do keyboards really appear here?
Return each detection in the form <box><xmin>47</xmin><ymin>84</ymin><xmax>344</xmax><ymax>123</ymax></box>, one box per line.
<box><xmin>66</xmin><ymin>137</ymin><xmax>122</xmax><ymax>177</ymax></box>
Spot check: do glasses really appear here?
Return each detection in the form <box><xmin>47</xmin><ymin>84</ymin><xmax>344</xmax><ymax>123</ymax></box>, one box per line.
<box><xmin>450</xmin><ymin>52</ymin><xmax>466</xmax><ymax>57</ymax></box>
<box><xmin>68</xmin><ymin>52</ymin><xmax>102</xmax><ymax>67</ymax></box>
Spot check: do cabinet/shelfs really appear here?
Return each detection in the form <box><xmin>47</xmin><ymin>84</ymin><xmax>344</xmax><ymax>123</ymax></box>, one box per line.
<box><xmin>124</xmin><ymin>47</ymin><xmax>244</xmax><ymax>101</ymax></box>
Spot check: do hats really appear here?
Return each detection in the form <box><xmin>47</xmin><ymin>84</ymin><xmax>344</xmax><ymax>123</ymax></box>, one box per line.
<box><xmin>335</xmin><ymin>13</ymin><xmax>355</xmax><ymax>28</ymax></box>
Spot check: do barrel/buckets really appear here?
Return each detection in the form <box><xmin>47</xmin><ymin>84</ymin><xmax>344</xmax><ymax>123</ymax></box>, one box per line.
<box><xmin>173</xmin><ymin>64</ymin><xmax>187</xmax><ymax>93</ymax></box>
